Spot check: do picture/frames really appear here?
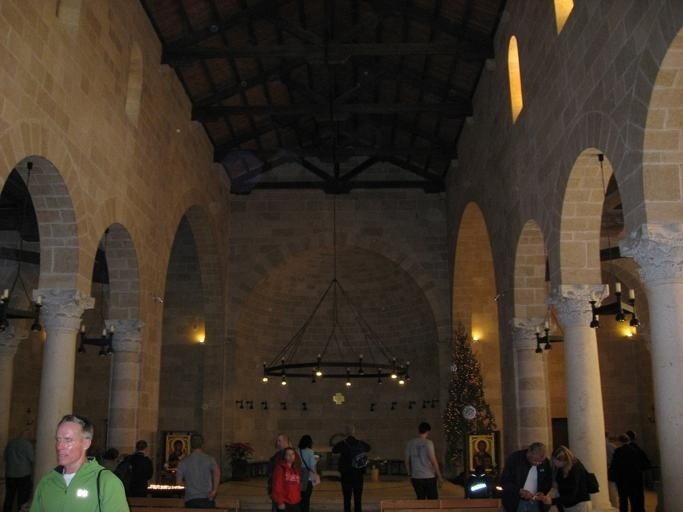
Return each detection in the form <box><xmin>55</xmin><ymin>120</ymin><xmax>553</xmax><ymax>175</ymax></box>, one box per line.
<box><xmin>464</xmin><ymin>431</ymin><xmax>501</xmax><ymax>472</ymax></box>
<box><xmin>161</xmin><ymin>431</ymin><xmax>196</xmax><ymax>472</ymax></box>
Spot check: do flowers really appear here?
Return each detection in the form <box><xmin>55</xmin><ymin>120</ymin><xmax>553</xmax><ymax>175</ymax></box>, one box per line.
<box><xmin>367</xmin><ymin>456</ymin><xmax>388</xmax><ymax>469</ymax></box>
<box><xmin>225</xmin><ymin>442</ymin><xmax>256</xmax><ymax>460</ymax></box>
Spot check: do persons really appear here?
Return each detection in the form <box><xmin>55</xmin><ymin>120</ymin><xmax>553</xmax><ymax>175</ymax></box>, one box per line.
<box><xmin>272</xmin><ymin>448</ymin><xmax>301</xmax><ymax>510</ymax></box>
<box><xmin>177</xmin><ymin>434</ymin><xmax>221</xmax><ymax>509</ymax></box>
<box><xmin>332</xmin><ymin>424</ymin><xmax>370</xmax><ymax>511</ymax></box>
<box><xmin>501</xmin><ymin>442</ymin><xmax>553</xmax><ymax>512</ymax></box>
<box><xmin>473</xmin><ymin>440</ymin><xmax>491</xmax><ymax>468</ymax></box>
<box><xmin>606</xmin><ymin>431</ymin><xmax>650</xmax><ymax>511</ymax></box>
<box><xmin>115</xmin><ymin>440</ymin><xmax>153</xmax><ymax>497</ymax></box>
<box><xmin>30</xmin><ymin>413</ymin><xmax>129</xmax><ymax>512</ymax></box>
<box><xmin>169</xmin><ymin>441</ymin><xmax>185</xmax><ymax>469</ymax></box>
<box><xmin>405</xmin><ymin>421</ymin><xmax>444</xmax><ymax>500</ymax></box>
<box><xmin>297</xmin><ymin>435</ymin><xmax>319</xmax><ymax>511</ymax></box>
<box><xmin>545</xmin><ymin>446</ymin><xmax>592</xmax><ymax>512</ymax></box>
<box><xmin>4</xmin><ymin>429</ymin><xmax>34</xmax><ymax>511</ymax></box>
<box><xmin>268</xmin><ymin>434</ymin><xmax>293</xmax><ymax>510</ymax></box>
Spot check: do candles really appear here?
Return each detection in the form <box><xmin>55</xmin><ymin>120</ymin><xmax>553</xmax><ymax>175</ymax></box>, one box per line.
<box><xmin>545</xmin><ymin>320</ymin><xmax>549</xmax><ymax>328</ymax></box>
<box><xmin>36</xmin><ymin>295</ymin><xmax>42</xmax><ymax>305</ymax></box>
<box><xmin>110</xmin><ymin>325</ymin><xmax>114</xmax><ymax>332</ymax></box>
<box><xmin>615</xmin><ymin>282</ymin><xmax>621</xmax><ymax>293</ymax></box>
<box><xmin>1</xmin><ymin>295</ymin><xmax>5</xmax><ymax>299</ymax></box>
<box><xmin>629</xmin><ymin>289</ymin><xmax>635</xmax><ymax>299</ymax></box>
<box><xmin>81</xmin><ymin>324</ymin><xmax>85</xmax><ymax>333</ymax></box>
<box><xmin>536</xmin><ymin>326</ymin><xmax>540</xmax><ymax>333</ymax></box>
<box><xmin>4</xmin><ymin>289</ymin><xmax>9</xmax><ymax>298</ymax></box>
<box><xmin>102</xmin><ymin>329</ymin><xmax>107</xmax><ymax>336</ymax></box>
<box><xmin>590</xmin><ymin>291</ymin><xmax>595</xmax><ymax>300</ymax></box>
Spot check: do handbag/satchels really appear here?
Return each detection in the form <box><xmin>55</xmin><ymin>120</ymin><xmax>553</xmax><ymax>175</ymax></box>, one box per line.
<box><xmin>309</xmin><ymin>471</ymin><xmax>321</xmax><ymax>487</ymax></box>
<box><xmin>587</xmin><ymin>472</ymin><xmax>599</xmax><ymax>494</ymax></box>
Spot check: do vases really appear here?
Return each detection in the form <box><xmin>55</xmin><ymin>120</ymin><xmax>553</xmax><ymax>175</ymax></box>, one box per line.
<box><xmin>371</xmin><ymin>469</ymin><xmax>380</xmax><ymax>480</ymax></box>
<box><xmin>232</xmin><ymin>459</ymin><xmax>249</xmax><ymax>481</ymax></box>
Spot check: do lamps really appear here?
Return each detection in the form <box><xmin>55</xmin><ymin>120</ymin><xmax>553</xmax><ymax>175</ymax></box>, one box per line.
<box><xmin>262</xmin><ymin>194</ymin><xmax>411</xmax><ymax>386</ymax></box>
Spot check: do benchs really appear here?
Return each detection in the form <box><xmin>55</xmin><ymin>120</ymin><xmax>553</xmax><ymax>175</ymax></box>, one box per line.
<box><xmin>126</xmin><ymin>497</ymin><xmax>240</xmax><ymax>512</ymax></box>
<box><xmin>379</xmin><ymin>498</ymin><xmax>505</xmax><ymax>512</ymax></box>
